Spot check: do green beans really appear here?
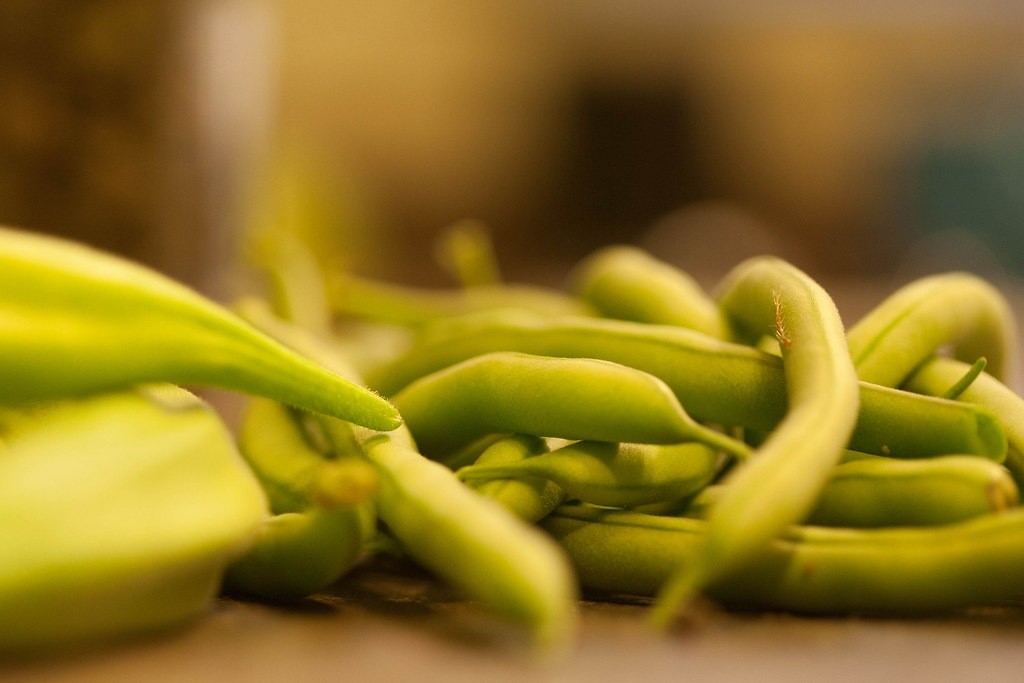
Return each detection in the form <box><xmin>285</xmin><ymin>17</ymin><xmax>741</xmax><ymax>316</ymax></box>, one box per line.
<box><xmin>0</xmin><ymin>228</ymin><xmax>1023</xmax><ymax>658</ymax></box>
